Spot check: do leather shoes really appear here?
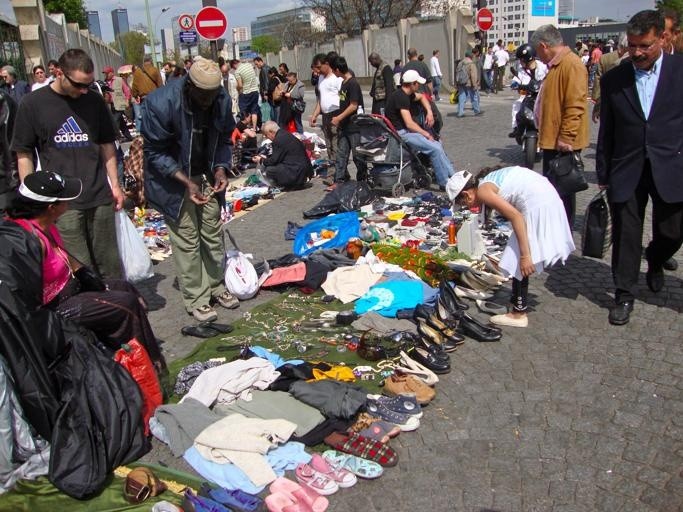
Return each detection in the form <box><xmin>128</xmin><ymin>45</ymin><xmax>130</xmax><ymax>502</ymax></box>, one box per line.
<box><xmin>645</xmin><ymin>262</ymin><xmax>664</xmax><ymax>292</ymax></box>
<box><xmin>609</xmin><ymin>300</ymin><xmax>634</xmax><ymax>325</ymax></box>
<box><xmin>414</xmin><ymin>254</ymin><xmax>529</xmax><ymax>374</ymax></box>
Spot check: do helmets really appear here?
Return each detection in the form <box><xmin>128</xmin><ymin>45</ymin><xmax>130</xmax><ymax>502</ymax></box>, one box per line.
<box><xmin>515</xmin><ymin>43</ymin><xmax>537</xmax><ymax>66</ymax></box>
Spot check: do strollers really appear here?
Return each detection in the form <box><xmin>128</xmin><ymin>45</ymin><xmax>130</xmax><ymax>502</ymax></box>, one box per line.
<box><xmin>345</xmin><ymin>113</ymin><xmax>434</xmax><ymax>199</ymax></box>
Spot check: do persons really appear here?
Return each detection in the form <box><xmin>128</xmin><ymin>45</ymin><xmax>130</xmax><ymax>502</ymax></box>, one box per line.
<box><xmin>574</xmin><ymin>10</ymin><xmax>683</xmax><ymax>325</ymax></box>
<box><xmin>508</xmin><ymin>25</ymin><xmax>590</xmax><ymax>227</ymax></box>
<box><xmin>458</xmin><ymin>39</ymin><xmax>509</xmax><ymax>118</ymax></box>
<box><xmin>367</xmin><ymin>48</ymin><xmax>457</xmax><ymax>191</ymax></box>
<box><xmin>446</xmin><ymin>164</ymin><xmax>577</xmax><ymax>327</ymax></box>
<box><xmin>309</xmin><ymin>51</ymin><xmax>366</xmax><ymax>189</ymax></box>
<box><xmin>1</xmin><ymin>48</ymin><xmax>131</xmax><ymax>293</ymax></box>
<box><xmin>130</xmin><ymin>57</ymin><xmax>313</xmax><ymax>320</ymax></box>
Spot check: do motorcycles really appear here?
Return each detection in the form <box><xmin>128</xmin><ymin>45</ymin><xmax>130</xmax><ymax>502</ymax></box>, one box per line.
<box><xmin>508</xmin><ymin>66</ymin><xmax>544</xmax><ymax>171</ymax></box>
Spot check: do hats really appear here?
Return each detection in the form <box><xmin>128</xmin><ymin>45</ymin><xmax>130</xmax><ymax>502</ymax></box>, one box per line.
<box><xmin>101</xmin><ymin>67</ymin><xmax>114</xmax><ymax>73</ymax></box>
<box><xmin>402</xmin><ymin>69</ymin><xmax>427</xmax><ymax>85</ymax></box>
<box><xmin>19</xmin><ymin>170</ymin><xmax>83</xmax><ymax>204</ymax></box>
<box><xmin>189</xmin><ymin>59</ymin><xmax>223</xmax><ymax>91</ymax></box>
<box><xmin>445</xmin><ymin>170</ymin><xmax>472</xmax><ymax>213</ymax></box>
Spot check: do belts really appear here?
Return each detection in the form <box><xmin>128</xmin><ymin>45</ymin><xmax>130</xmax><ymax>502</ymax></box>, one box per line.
<box><xmin>123</xmin><ymin>467</ymin><xmax>167</xmax><ymax>503</ymax></box>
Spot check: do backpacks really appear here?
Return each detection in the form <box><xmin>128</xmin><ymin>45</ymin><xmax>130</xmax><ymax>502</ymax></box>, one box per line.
<box><xmin>456</xmin><ymin>58</ymin><xmax>476</xmax><ymax>86</ymax></box>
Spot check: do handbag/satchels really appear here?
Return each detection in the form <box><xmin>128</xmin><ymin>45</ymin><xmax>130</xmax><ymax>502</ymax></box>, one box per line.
<box><xmin>580</xmin><ymin>199</ymin><xmax>612</xmax><ymax>258</ymax></box>
<box><xmin>289</xmin><ymin>98</ymin><xmax>306</xmax><ymax>112</ymax></box>
<box><xmin>272</xmin><ymin>83</ymin><xmax>287</xmax><ymax>102</ymax></box>
<box><xmin>549</xmin><ymin>152</ymin><xmax>588</xmax><ymax>196</ymax></box>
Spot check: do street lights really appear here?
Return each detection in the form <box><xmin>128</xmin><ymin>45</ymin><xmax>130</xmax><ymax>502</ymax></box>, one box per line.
<box><xmin>154</xmin><ymin>7</ymin><xmax>170</xmax><ymax>63</ymax></box>
<box><xmin>281</xmin><ymin>14</ymin><xmax>304</xmax><ymax>49</ymax></box>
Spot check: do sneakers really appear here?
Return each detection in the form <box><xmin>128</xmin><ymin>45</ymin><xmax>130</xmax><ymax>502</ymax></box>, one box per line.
<box><xmin>265</xmin><ymin>431</ymin><xmax>399</xmax><ymax>511</ymax></box>
<box><xmin>215</xmin><ymin>291</ymin><xmax>240</xmax><ymax>309</ymax></box>
<box><xmin>152</xmin><ymin>481</ymin><xmax>268</xmax><ymax>512</ymax></box>
<box><xmin>347</xmin><ymin>348</ymin><xmax>440</xmax><ymax>444</ymax></box>
<box><xmin>664</xmin><ymin>257</ymin><xmax>678</xmax><ymax>271</ymax></box>
<box><xmin>475</xmin><ymin>110</ymin><xmax>486</xmax><ymax>116</ymax></box>
<box><xmin>191</xmin><ymin>303</ymin><xmax>217</xmax><ymax>322</ymax></box>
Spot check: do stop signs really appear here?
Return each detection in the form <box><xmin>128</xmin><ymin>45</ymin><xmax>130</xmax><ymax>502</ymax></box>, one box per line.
<box><xmin>193</xmin><ymin>5</ymin><xmax>228</xmax><ymax>41</ymax></box>
<box><xmin>476</xmin><ymin>8</ymin><xmax>493</xmax><ymax>32</ymax></box>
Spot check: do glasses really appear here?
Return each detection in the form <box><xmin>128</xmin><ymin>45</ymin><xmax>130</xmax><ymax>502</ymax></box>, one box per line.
<box><xmin>61</xmin><ymin>69</ymin><xmax>95</xmax><ymax>90</ymax></box>
<box><xmin>624</xmin><ymin>42</ymin><xmax>656</xmax><ymax>52</ymax></box>
